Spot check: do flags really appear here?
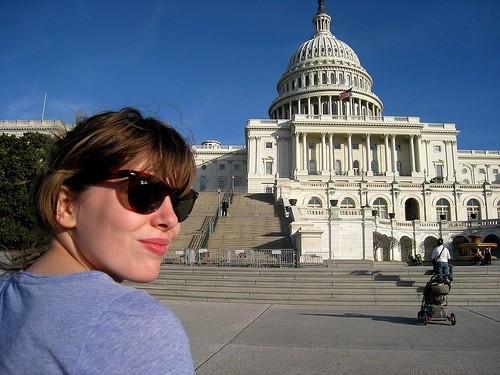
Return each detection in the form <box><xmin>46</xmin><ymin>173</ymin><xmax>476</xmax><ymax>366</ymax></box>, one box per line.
<box><xmin>339</xmin><ymin>88</ymin><xmax>352</xmax><ymax>99</ymax></box>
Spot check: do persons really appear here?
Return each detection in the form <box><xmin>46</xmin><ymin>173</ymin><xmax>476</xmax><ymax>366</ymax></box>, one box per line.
<box><xmin>484</xmin><ymin>250</ymin><xmax>492</xmax><ymax>265</ymax></box>
<box><xmin>222</xmin><ymin>199</ymin><xmax>229</xmax><ymax>217</ymax></box>
<box><xmin>431</xmin><ymin>239</ymin><xmax>451</xmax><ymax>281</ymax></box>
<box><xmin>0</xmin><ymin>106</ymin><xmax>200</xmax><ymax>374</ymax></box>
<box><xmin>408</xmin><ymin>253</ymin><xmax>417</xmax><ymax>266</ymax></box>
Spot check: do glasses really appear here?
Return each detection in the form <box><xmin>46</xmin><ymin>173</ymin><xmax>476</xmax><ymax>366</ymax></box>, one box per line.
<box><xmin>83</xmin><ymin>169</ymin><xmax>200</xmax><ymax>222</ymax></box>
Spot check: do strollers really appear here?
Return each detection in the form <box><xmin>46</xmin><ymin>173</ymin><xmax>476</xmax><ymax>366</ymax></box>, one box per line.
<box><xmin>417</xmin><ymin>274</ymin><xmax>457</xmax><ymax>325</ymax></box>
